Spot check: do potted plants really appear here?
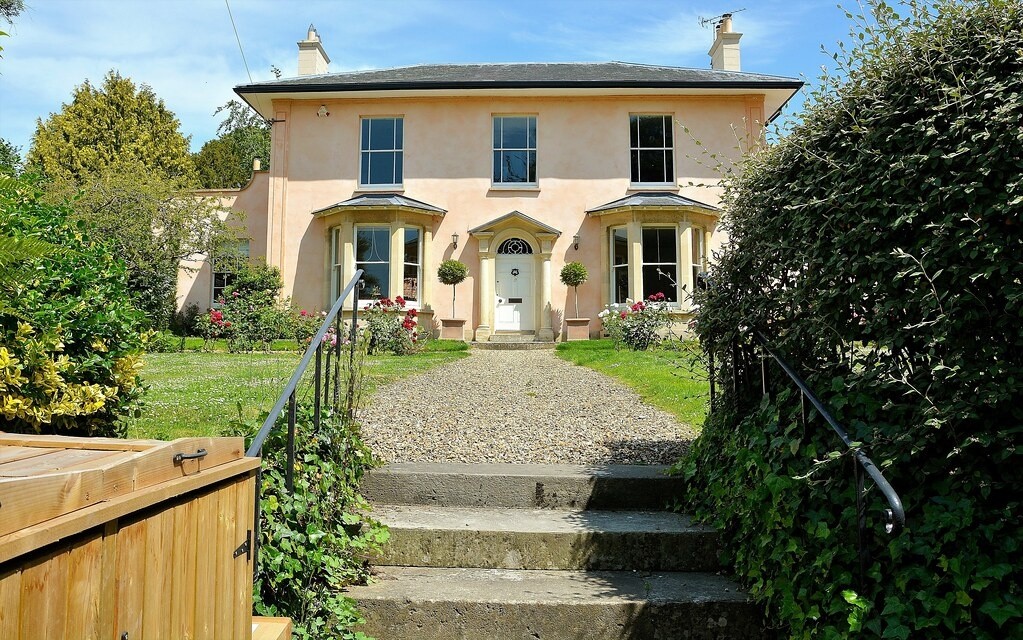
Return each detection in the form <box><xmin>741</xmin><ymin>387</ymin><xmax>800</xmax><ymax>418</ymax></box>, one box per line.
<box><xmin>437</xmin><ymin>259</ymin><xmax>471</xmax><ymax>340</ymax></box>
<box><xmin>560</xmin><ymin>260</ymin><xmax>591</xmax><ymax>340</ymax></box>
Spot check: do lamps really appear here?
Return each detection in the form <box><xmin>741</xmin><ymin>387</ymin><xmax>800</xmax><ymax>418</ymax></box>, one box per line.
<box><xmin>572</xmin><ymin>233</ymin><xmax>580</xmax><ymax>250</ymax></box>
<box><xmin>452</xmin><ymin>232</ymin><xmax>460</xmax><ymax>249</ymax></box>
